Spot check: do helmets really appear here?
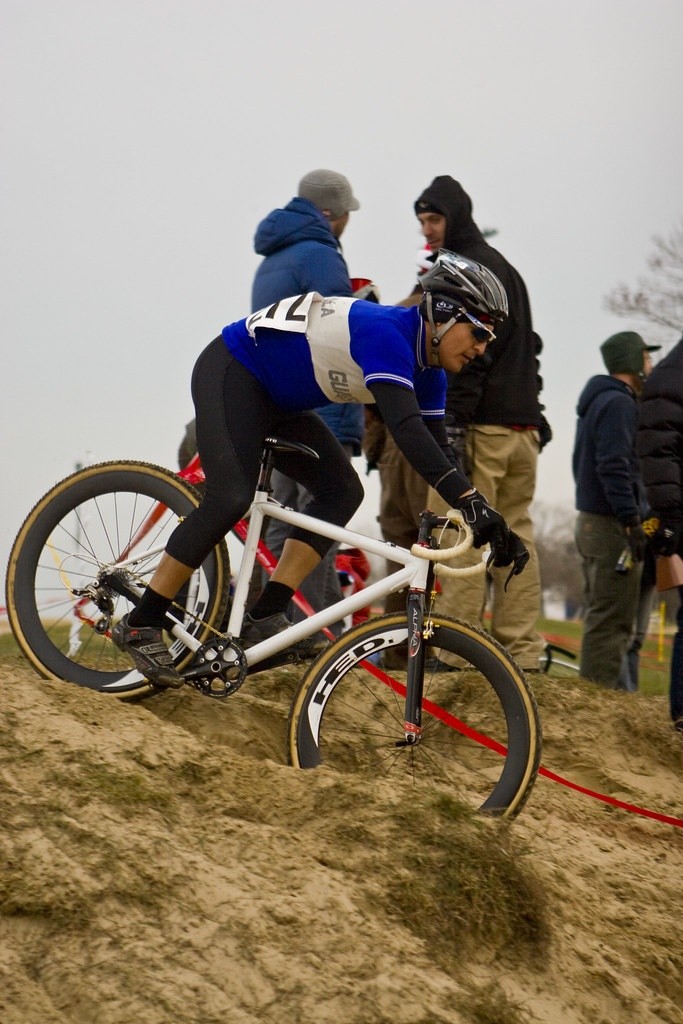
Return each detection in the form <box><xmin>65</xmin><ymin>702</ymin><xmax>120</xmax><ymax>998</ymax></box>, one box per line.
<box><xmin>416</xmin><ymin>247</ymin><xmax>509</xmax><ymax>323</ymax></box>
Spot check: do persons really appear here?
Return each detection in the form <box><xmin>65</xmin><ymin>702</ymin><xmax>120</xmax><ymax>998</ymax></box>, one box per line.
<box><xmin>573</xmin><ymin>330</ymin><xmax>683</xmax><ymax>730</ymax></box>
<box><xmin>178</xmin><ymin>169</ymin><xmax>552</xmax><ymax>673</ymax></box>
<box><xmin>113</xmin><ymin>252</ymin><xmax>528</xmax><ymax>690</ymax></box>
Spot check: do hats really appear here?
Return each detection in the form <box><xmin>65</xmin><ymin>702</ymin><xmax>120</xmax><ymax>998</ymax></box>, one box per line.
<box><xmin>416</xmin><ymin>200</ymin><xmax>444</xmax><ymax>217</ymax></box>
<box><xmin>600</xmin><ymin>331</ymin><xmax>661</xmax><ymax>375</ymax></box>
<box><xmin>297</xmin><ymin>168</ymin><xmax>360</xmax><ymax>218</ymax></box>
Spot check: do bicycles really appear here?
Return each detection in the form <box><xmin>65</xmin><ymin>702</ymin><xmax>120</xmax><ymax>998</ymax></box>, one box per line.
<box><xmin>3</xmin><ymin>430</ymin><xmax>544</xmax><ymax>823</ymax></box>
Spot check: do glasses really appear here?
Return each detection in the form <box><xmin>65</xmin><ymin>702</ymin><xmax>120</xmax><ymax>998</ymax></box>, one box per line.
<box><xmin>457</xmin><ymin>307</ymin><xmax>496</xmax><ymax>344</ymax></box>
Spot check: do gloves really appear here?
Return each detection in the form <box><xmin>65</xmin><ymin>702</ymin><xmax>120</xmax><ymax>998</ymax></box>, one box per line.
<box><xmin>489</xmin><ymin>523</ymin><xmax>516</xmax><ymax>568</ymax></box>
<box><xmin>457</xmin><ymin>487</ymin><xmax>511</xmax><ymax>550</ymax></box>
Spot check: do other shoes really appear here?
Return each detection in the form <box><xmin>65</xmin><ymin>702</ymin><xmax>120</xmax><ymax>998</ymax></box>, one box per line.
<box><xmin>383</xmin><ymin>654</ymin><xmax>459</xmax><ymax>673</ymax></box>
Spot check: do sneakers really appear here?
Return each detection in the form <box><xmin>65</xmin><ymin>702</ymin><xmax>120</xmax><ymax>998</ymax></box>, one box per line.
<box><xmin>241</xmin><ymin>613</ymin><xmax>315</xmax><ymax>657</ymax></box>
<box><xmin>112</xmin><ymin>613</ymin><xmax>184</xmax><ymax>689</ymax></box>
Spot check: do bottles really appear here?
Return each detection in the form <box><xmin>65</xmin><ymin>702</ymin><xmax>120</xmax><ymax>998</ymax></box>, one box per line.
<box><xmin>614</xmin><ymin>520</ymin><xmax>647</xmax><ymax>575</ymax></box>
